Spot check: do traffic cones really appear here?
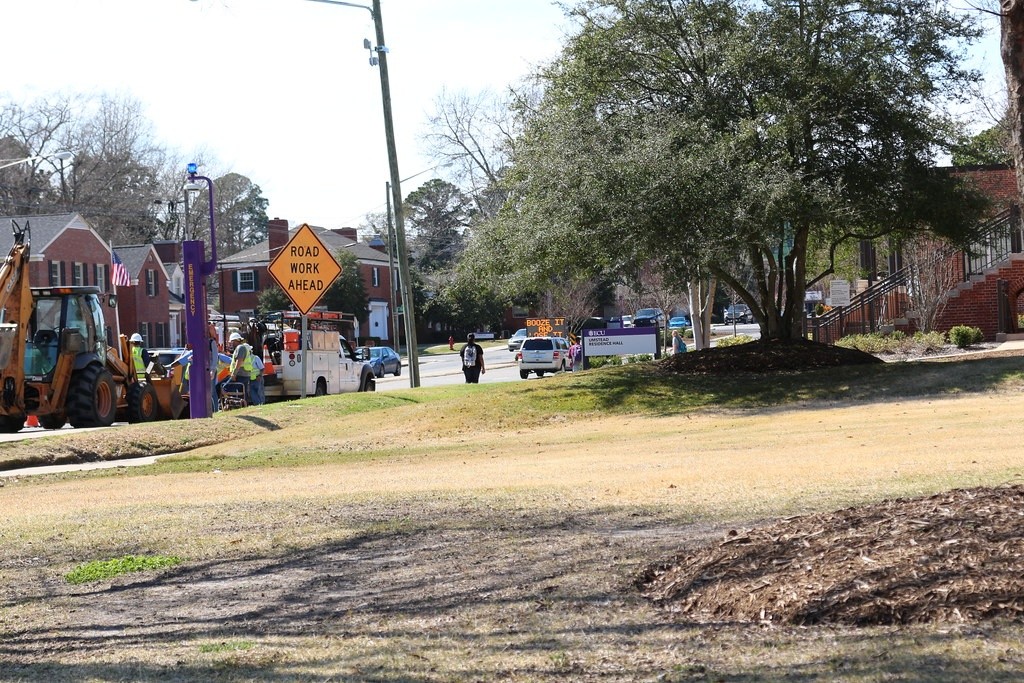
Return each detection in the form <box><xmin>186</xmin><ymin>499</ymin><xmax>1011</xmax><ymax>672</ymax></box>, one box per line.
<box><xmin>24</xmin><ymin>415</ymin><xmax>40</xmax><ymax>428</ymax></box>
<box><xmin>261</xmin><ymin>344</ymin><xmax>276</xmax><ymax>374</ymax></box>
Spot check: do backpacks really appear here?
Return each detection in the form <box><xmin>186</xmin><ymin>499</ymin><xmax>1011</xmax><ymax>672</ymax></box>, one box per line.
<box><xmin>675</xmin><ymin>336</ymin><xmax>687</xmax><ymax>353</ymax></box>
<box><xmin>573</xmin><ymin>344</ymin><xmax>582</xmax><ymax>362</ymax></box>
<box><xmin>464</xmin><ymin>344</ymin><xmax>477</xmax><ymax>368</ymax></box>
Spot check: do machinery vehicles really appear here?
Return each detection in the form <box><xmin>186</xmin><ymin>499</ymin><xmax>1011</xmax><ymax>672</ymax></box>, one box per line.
<box><xmin>0</xmin><ymin>220</ymin><xmax>188</xmax><ymax>434</ymax></box>
<box><xmin>222</xmin><ymin>311</ymin><xmax>376</xmax><ymax>405</ymax></box>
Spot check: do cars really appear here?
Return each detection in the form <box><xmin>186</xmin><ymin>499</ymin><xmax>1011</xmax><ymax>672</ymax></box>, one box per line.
<box><xmin>145</xmin><ymin>348</ymin><xmax>233</xmax><ymax>398</ymax></box>
<box><xmin>621</xmin><ymin>314</ymin><xmax>633</xmax><ymax>327</ymax></box>
<box><xmin>354</xmin><ymin>346</ymin><xmax>402</xmax><ymax>377</ymax></box>
<box><xmin>507</xmin><ymin>328</ymin><xmax>528</xmax><ymax>352</ymax></box>
<box><xmin>723</xmin><ymin>305</ymin><xmax>756</xmax><ymax>325</ymax></box>
<box><xmin>667</xmin><ymin>317</ymin><xmax>691</xmax><ymax>329</ymax></box>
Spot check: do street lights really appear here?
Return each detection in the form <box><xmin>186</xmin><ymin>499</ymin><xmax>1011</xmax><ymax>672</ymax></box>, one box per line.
<box><xmin>384</xmin><ymin>162</ymin><xmax>452</xmax><ymax>354</ymax></box>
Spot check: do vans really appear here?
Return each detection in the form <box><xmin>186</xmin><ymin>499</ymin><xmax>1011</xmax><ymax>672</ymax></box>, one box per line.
<box><xmin>632</xmin><ymin>307</ymin><xmax>669</xmax><ymax>332</ymax></box>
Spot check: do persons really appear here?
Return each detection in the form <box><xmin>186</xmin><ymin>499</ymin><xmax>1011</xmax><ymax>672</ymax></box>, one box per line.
<box><xmin>460</xmin><ymin>333</ymin><xmax>485</xmax><ymax>383</ymax></box>
<box><xmin>170</xmin><ymin>324</ymin><xmax>265</xmax><ymax>412</ymax></box>
<box><xmin>568</xmin><ymin>337</ymin><xmax>582</xmax><ymax>372</ymax></box>
<box><xmin>128</xmin><ymin>333</ymin><xmax>151</xmax><ymax>384</ymax></box>
<box><xmin>672</xmin><ymin>330</ymin><xmax>687</xmax><ymax>354</ymax></box>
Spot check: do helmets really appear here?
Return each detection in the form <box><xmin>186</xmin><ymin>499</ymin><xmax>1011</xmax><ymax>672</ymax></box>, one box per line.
<box><xmin>129</xmin><ymin>333</ymin><xmax>143</xmax><ymax>342</ymax></box>
<box><xmin>228</xmin><ymin>332</ymin><xmax>244</xmax><ymax>343</ymax></box>
<box><xmin>243</xmin><ymin>343</ymin><xmax>253</xmax><ymax>351</ymax></box>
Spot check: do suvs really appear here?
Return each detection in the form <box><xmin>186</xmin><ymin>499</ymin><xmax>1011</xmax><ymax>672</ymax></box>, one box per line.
<box><xmin>515</xmin><ymin>336</ymin><xmax>572</xmax><ymax>379</ymax></box>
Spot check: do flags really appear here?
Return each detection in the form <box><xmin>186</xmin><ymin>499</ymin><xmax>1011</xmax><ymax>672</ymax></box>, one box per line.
<box><xmin>112</xmin><ymin>249</ymin><xmax>131</xmax><ymax>288</ymax></box>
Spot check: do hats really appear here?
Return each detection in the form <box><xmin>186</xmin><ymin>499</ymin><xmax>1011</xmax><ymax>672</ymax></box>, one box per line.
<box><xmin>467</xmin><ymin>333</ymin><xmax>475</xmax><ymax>338</ymax></box>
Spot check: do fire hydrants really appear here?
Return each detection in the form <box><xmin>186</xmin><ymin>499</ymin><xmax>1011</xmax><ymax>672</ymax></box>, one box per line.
<box><xmin>448</xmin><ymin>335</ymin><xmax>453</xmax><ymax>349</ymax></box>
